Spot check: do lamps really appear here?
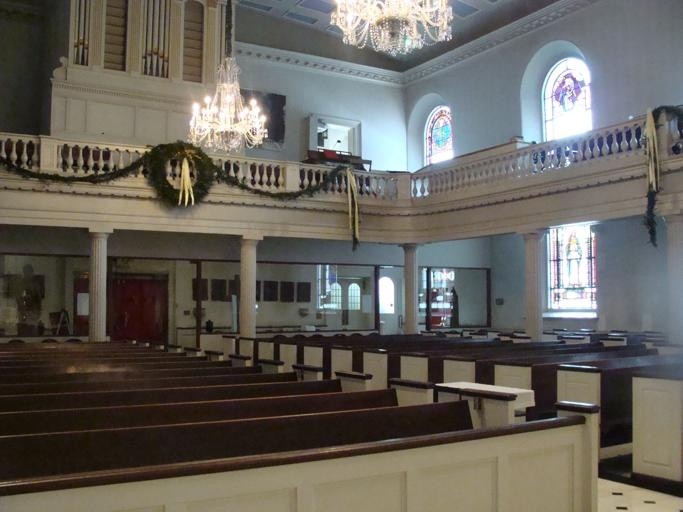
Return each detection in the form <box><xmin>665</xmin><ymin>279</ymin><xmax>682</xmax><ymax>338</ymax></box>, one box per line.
<box><xmin>188</xmin><ymin>0</ymin><xmax>268</xmax><ymax>153</ymax></box>
<box><xmin>330</xmin><ymin>1</ymin><xmax>453</xmax><ymax>57</ymax></box>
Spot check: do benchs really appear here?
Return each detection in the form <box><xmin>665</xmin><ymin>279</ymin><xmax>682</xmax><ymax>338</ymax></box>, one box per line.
<box><xmin>0</xmin><ymin>340</ymin><xmax>150</xmax><ymax>348</ymax></box>
<box><xmin>494</xmin><ymin>348</ymin><xmax>661</xmax><ymax>422</ymax></box>
<box><xmin>0</xmin><ymin>381</ymin><xmax>535</xmax><ymax>480</ymax></box>
<box><xmin>279</xmin><ymin>336</ymin><xmax>475</xmax><ymax>372</ymax></box>
<box><xmin>420</xmin><ymin>327</ymin><xmax>533</xmax><ymax>341</ymax></box>
<box><xmin>0</xmin><ymin>345</ymin><xmax>182</xmax><ymax>360</ymax></box>
<box><xmin>0</xmin><ymin>377</ymin><xmax>435</xmax><ymax>435</ymax></box>
<box><xmin>543</xmin><ymin>327</ymin><xmax>679</xmax><ymax>343</ymax></box>
<box><xmin>239</xmin><ymin>337</ymin><xmax>256</xmax><ymax>365</ymax></box>
<box><xmin>0</xmin><ymin>359</ymin><xmax>285</xmax><ymax>382</ymax></box>
<box><xmin>222</xmin><ymin>335</ymin><xmax>238</xmax><ymax>360</ymax></box>
<box><xmin>0</xmin><ymin>348</ymin><xmax>225</xmax><ymax>365</ymax></box>
<box><xmin>0</xmin><ymin>370</ymin><xmax>373</xmax><ymax>411</ymax></box>
<box><xmin>400</xmin><ymin>344</ymin><xmax>608</xmax><ymax>381</ymax></box>
<box><xmin>631</xmin><ymin>370</ymin><xmax>683</xmax><ymax>484</ymax></box>
<box><xmin>1</xmin><ymin>399</ymin><xmax>601</xmax><ymax>512</ymax></box>
<box><xmin>257</xmin><ymin>336</ymin><xmax>279</xmax><ymax>361</ymax></box>
<box><xmin>0</xmin><ymin>355</ymin><xmax>254</xmax><ymax>371</ymax></box>
<box><xmin>442</xmin><ymin>344</ymin><xmax>643</xmax><ymax>384</ymax></box>
<box><xmin>0</xmin><ymin>344</ymin><xmax>164</xmax><ymax>356</ymax></box>
<box><xmin>0</xmin><ymin>345</ymin><xmax>204</xmax><ymax>359</ymax></box>
<box><xmin>554</xmin><ymin>352</ymin><xmax>683</xmax><ymax>460</ymax></box>
<box><xmin>303</xmin><ymin>337</ymin><xmax>501</xmax><ymax>379</ymax></box>
<box><xmin>329</xmin><ymin>339</ymin><xmax>513</xmax><ymax>379</ymax></box>
<box><xmin>362</xmin><ymin>342</ymin><xmax>566</xmax><ymax>387</ymax></box>
<box><xmin>0</xmin><ymin>365</ymin><xmax>324</xmax><ymax>397</ymax></box>
